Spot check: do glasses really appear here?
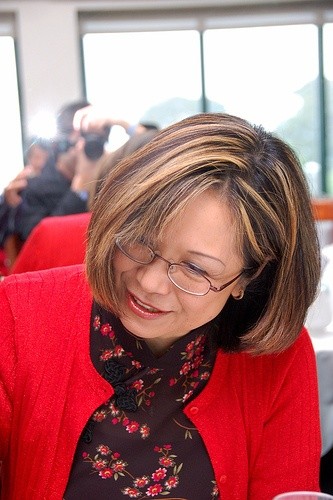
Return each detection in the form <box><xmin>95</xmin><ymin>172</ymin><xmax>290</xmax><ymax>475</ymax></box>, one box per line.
<box><xmin>112</xmin><ymin>234</ymin><xmax>246</xmax><ymax>297</ymax></box>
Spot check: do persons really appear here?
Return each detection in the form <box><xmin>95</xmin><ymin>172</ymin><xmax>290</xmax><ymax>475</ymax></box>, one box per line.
<box><xmin>0</xmin><ymin>101</ymin><xmax>158</xmax><ymax>276</ymax></box>
<box><xmin>0</xmin><ymin>114</ymin><xmax>322</xmax><ymax>500</ymax></box>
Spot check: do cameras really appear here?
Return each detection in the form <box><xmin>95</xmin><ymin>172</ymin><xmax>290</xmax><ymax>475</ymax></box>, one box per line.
<box><xmin>81</xmin><ymin>131</ymin><xmax>105</xmax><ymax>160</ymax></box>
